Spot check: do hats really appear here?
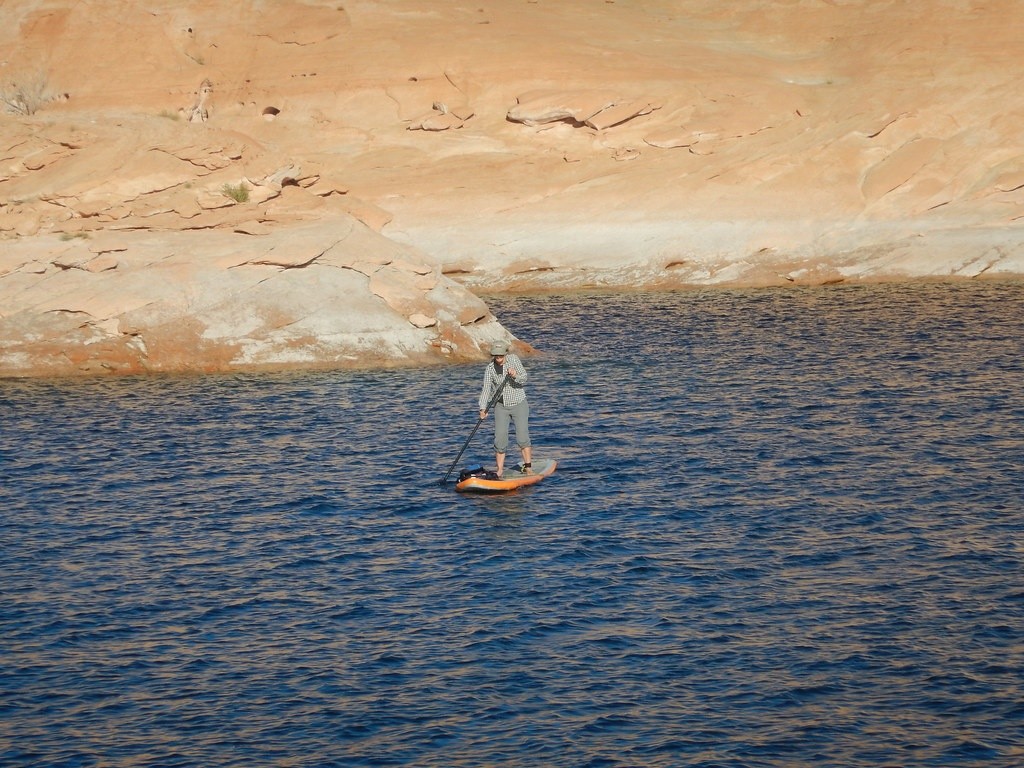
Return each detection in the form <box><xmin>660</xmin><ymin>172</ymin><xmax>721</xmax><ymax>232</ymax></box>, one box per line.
<box><xmin>490</xmin><ymin>340</ymin><xmax>507</xmax><ymax>356</ymax></box>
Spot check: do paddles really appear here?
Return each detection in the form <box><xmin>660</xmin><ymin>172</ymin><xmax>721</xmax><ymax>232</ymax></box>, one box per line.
<box><xmin>442</xmin><ymin>373</ymin><xmax>511</xmax><ymax>484</ymax></box>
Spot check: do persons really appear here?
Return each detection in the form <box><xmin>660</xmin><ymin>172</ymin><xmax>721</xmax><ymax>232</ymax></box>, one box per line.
<box><xmin>480</xmin><ymin>340</ymin><xmax>534</xmax><ymax>477</ymax></box>
